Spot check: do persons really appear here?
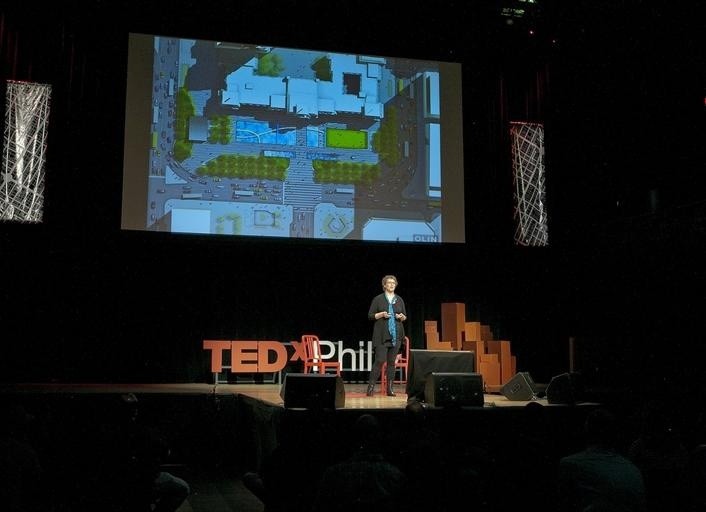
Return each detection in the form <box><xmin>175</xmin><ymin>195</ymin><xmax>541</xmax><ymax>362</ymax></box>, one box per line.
<box><xmin>1</xmin><ymin>396</ymin><xmax>706</xmax><ymax>511</ymax></box>
<box><xmin>366</xmin><ymin>275</ymin><xmax>407</xmax><ymax>397</ymax></box>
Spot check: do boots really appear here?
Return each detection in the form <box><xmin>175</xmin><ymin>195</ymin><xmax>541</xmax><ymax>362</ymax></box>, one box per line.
<box><xmin>386</xmin><ymin>380</ymin><xmax>395</xmax><ymax>396</ymax></box>
<box><xmin>367</xmin><ymin>380</ymin><xmax>376</xmax><ymax>396</ymax></box>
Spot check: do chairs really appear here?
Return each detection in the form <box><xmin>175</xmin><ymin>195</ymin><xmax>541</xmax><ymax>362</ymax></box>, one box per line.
<box><xmin>381</xmin><ymin>336</ymin><xmax>409</xmax><ymax>395</ymax></box>
<box><xmin>302</xmin><ymin>335</ymin><xmax>340</xmax><ymax>376</ymax></box>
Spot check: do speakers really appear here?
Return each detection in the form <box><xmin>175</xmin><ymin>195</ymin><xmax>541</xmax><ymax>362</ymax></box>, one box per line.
<box><xmin>500</xmin><ymin>372</ymin><xmax>536</xmax><ymax>401</ymax></box>
<box><xmin>546</xmin><ymin>370</ymin><xmax>589</xmax><ymax>404</ymax></box>
<box><xmin>283</xmin><ymin>372</ymin><xmax>346</xmax><ymax>409</ymax></box>
<box><xmin>424</xmin><ymin>371</ymin><xmax>485</xmax><ymax>407</ymax></box>
